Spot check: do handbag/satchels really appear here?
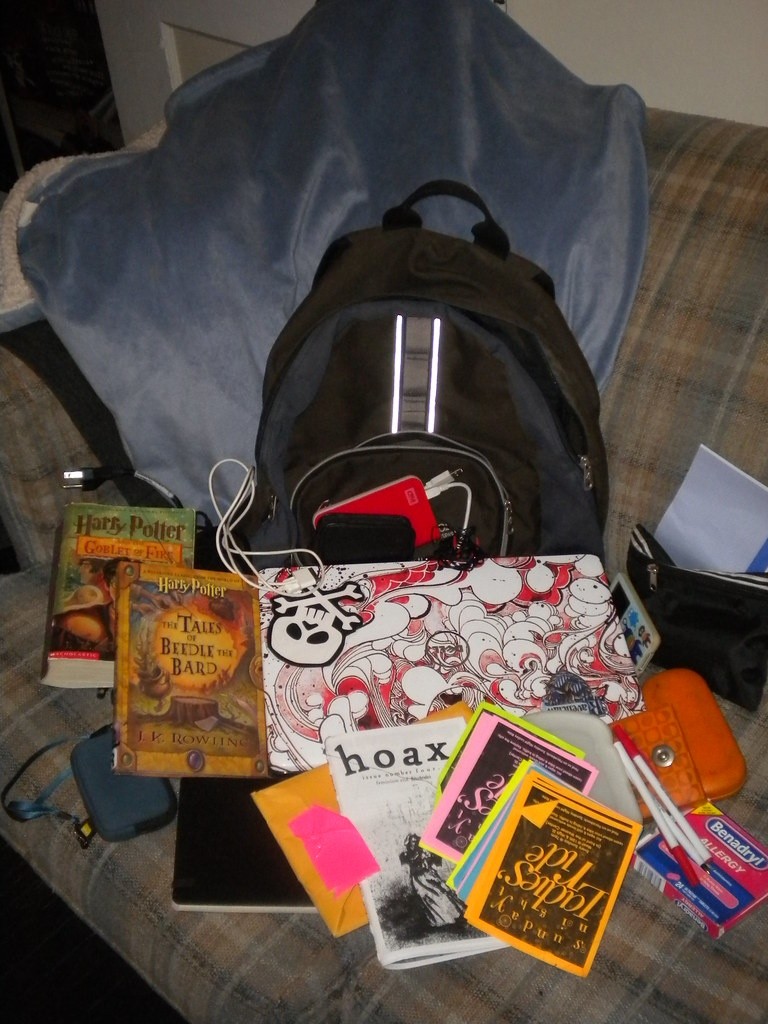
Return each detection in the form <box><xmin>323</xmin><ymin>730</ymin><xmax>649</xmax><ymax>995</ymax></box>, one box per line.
<box><xmin>626</xmin><ymin>523</ymin><xmax>768</xmax><ymax>712</ymax></box>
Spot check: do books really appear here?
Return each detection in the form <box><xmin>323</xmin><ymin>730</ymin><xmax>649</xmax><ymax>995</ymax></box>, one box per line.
<box><xmin>39</xmin><ymin>501</ymin><xmax>195</xmax><ymax>689</ymax></box>
<box><xmin>252</xmin><ymin>700</ymin><xmax>644</xmax><ymax>977</ymax></box>
<box><xmin>654</xmin><ymin>444</ymin><xmax>768</xmax><ymax>573</ymax></box>
<box><xmin>113</xmin><ymin>562</ymin><xmax>268</xmax><ymax>777</ymax></box>
<box><xmin>171</xmin><ymin>777</ymin><xmax>319</xmax><ymax>913</ymax></box>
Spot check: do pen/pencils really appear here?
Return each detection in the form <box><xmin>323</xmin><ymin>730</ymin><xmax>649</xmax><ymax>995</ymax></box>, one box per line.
<box><xmin>612</xmin><ymin>724</ymin><xmax>714</xmax><ymax>888</ymax></box>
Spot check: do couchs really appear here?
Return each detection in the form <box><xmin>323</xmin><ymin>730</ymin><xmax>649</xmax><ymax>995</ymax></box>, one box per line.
<box><xmin>0</xmin><ymin>107</ymin><xmax>768</xmax><ymax>1024</ymax></box>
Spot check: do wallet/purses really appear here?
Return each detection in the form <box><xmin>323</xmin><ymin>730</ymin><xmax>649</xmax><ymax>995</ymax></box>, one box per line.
<box><xmin>608</xmin><ymin>706</ymin><xmax>707</xmax><ymax>824</ymax></box>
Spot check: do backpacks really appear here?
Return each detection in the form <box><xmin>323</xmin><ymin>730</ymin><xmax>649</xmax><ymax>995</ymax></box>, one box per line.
<box><xmin>245</xmin><ymin>181</ymin><xmax>609</xmax><ymax>579</ymax></box>
